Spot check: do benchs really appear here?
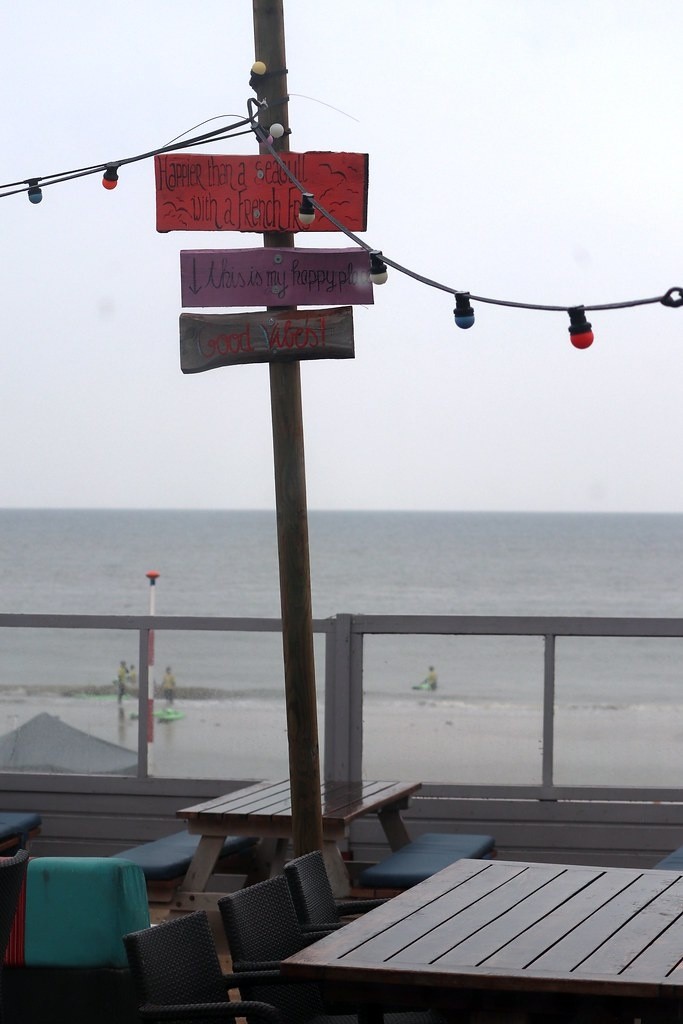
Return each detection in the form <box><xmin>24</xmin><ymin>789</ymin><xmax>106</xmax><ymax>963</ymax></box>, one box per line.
<box><xmin>356</xmin><ymin>833</ymin><xmax>496</xmax><ymax>893</ymax></box>
<box><xmin>111</xmin><ymin>831</ymin><xmax>260</xmax><ymax>906</ymax></box>
<box><xmin>0</xmin><ymin>810</ymin><xmax>43</xmax><ymax>853</ymax></box>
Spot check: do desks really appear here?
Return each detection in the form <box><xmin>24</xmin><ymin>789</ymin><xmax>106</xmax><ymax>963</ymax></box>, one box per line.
<box><xmin>166</xmin><ymin>780</ymin><xmax>423</xmax><ymax>925</ymax></box>
<box><xmin>281</xmin><ymin>857</ymin><xmax>683</xmax><ymax>1024</ymax></box>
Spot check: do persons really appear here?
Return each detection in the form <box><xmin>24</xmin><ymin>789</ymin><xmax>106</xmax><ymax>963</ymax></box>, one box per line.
<box><xmin>127</xmin><ymin>665</ymin><xmax>137</xmax><ymax>683</ymax></box>
<box><xmin>422</xmin><ymin>666</ymin><xmax>437</xmax><ymax>689</ymax></box>
<box><xmin>157</xmin><ymin>667</ymin><xmax>177</xmax><ymax>700</ymax></box>
<box><xmin>118</xmin><ymin>661</ymin><xmax>130</xmax><ymax>694</ymax></box>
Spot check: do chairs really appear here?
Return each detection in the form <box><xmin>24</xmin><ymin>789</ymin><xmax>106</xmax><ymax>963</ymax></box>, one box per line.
<box><xmin>123</xmin><ymin>909</ymin><xmax>307</xmax><ymax>1024</ymax></box>
<box><xmin>217</xmin><ymin>875</ymin><xmax>429</xmax><ymax>1024</ymax></box>
<box><xmin>284</xmin><ymin>849</ymin><xmax>393</xmax><ymax>928</ymax></box>
<box><xmin>0</xmin><ymin>849</ymin><xmax>29</xmax><ymax>969</ymax></box>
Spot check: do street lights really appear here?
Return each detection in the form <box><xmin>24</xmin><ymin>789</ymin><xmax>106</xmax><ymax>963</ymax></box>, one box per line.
<box><xmin>140</xmin><ymin>568</ymin><xmax>160</xmax><ymax>743</ymax></box>
